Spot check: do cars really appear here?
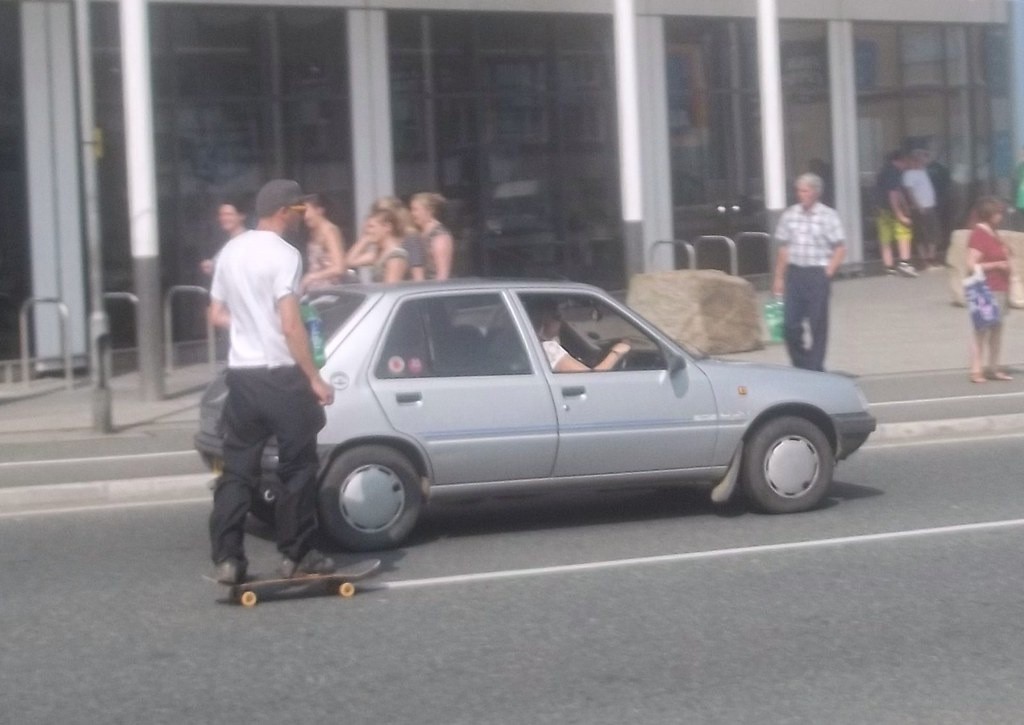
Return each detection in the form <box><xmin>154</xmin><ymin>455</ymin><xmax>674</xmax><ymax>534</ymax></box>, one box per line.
<box><xmin>195</xmin><ymin>280</ymin><xmax>877</xmax><ymax>550</ymax></box>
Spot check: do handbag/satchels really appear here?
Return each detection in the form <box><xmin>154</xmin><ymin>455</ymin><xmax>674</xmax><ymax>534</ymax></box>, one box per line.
<box><xmin>962</xmin><ymin>265</ymin><xmax>1000</xmax><ymax>331</ymax></box>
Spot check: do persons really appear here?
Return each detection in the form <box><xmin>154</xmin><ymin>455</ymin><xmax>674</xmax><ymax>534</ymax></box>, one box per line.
<box><xmin>873</xmin><ymin>134</ymin><xmax>948</xmax><ymax>276</ymax></box>
<box><xmin>966</xmin><ymin>195</ymin><xmax>1015</xmax><ymax>384</ymax></box>
<box><xmin>808</xmin><ymin>155</ymin><xmax>834</xmax><ymax>209</ymax></box>
<box><xmin>524</xmin><ymin>295</ymin><xmax>632</xmax><ymax>373</ymax></box>
<box><xmin>776</xmin><ymin>172</ymin><xmax>847</xmax><ymax>370</ymax></box>
<box><xmin>209</xmin><ymin>176</ymin><xmax>335</xmax><ymax>583</ymax></box>
<box><xmin>303</xmin><ymin>191</ymin><xmax>348</xmax><ymax>284</ymax></box>
<box><xmin>198</xmin><ymin>198</ymin><xmax>252</xmax><ymax>277</ymax></box>
<box><xmin>346</xmin><ymin>190</ymin><xmax>457</xmax><ymax>284</ymax></box>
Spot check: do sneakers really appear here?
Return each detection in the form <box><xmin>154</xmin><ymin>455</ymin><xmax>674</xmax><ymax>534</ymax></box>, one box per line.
<box><xmin>217</xmin><ymin>562</ymin><xmax>239</xmax><ymax>583</ymax></box>
<box><xmin>281</xmin><ymin>549</ymin><xmax>335</xmax><ymax>578</ymax></box>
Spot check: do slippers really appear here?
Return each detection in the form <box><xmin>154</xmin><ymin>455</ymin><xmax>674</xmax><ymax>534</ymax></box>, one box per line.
<box><xmin>969</xmin><ymin>372</ymin><xmax>986</xmax><ymax>384</ymax></box>
<box><xmin>985</xmin><ymin>370</ymin><xmax>1012</xmax><ymax>381</ymax></box>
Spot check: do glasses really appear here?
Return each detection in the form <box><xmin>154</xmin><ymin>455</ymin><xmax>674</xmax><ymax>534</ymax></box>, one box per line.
<box><xmin>547</xmin><ymin>314</ymin><xmax>563</xmax><ymax>322</ymax></box>
<box><xmin>284</xmin><ymin>204</ymin><xmax>308</xmax><ymax>215</ymax></box>
<box><xmin>994</xmin><ymin>209</ymin><xmax>1003</xmax><ymax>215</ymax></box>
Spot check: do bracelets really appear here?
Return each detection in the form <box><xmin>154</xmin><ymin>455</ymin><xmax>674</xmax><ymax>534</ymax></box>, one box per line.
<box><xmin>609</xmin><ymin>349</ymin><xmax>622</xmax><ymax>358</ymax></box>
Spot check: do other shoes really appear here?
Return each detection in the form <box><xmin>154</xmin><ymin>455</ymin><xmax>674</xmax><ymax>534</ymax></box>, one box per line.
<box><xmin>897</xmin><ymin>260</ymin><xmax>917</xmax><ymax>276</ymax></box>
<box><xmin>883</xmin><ymin>264</ymin><xmax>897</xmax><ymax>274</ymax></box>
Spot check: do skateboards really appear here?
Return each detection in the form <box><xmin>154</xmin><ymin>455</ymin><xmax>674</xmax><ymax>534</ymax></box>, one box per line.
<box><xmin>200</xmin><ymin>557</ymin><xmax>382</xmax><ymax>609</ymax></box>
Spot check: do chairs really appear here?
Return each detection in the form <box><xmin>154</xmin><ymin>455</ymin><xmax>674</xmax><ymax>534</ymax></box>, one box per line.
<box><xmin>483</xmin><ymin>310</ymin><xmax>533</xmax><ymax>375</ymax></box>
<box><xmin>438</xmin><ymin>326</ymin><xmax>484</xmax><ymax>375</ymax></box>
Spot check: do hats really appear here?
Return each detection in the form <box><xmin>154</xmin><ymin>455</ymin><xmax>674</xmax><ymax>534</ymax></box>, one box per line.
<box><xmin>256</xmin><ymin>181</ymin><xmax>318</xmax><ymax>215</ymax></box>
